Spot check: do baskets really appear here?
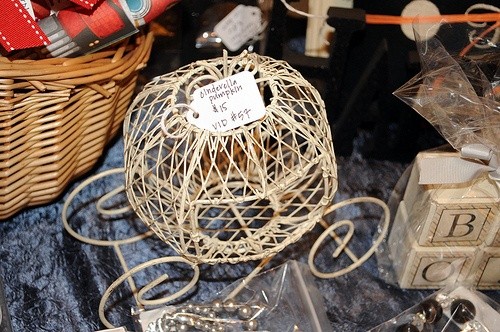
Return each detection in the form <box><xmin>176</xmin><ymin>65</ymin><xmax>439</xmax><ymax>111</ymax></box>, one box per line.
<box><xmin>0</xmin><ymin>19</ymin><xmax>155</xmax><ymax>221</ymax></box>
